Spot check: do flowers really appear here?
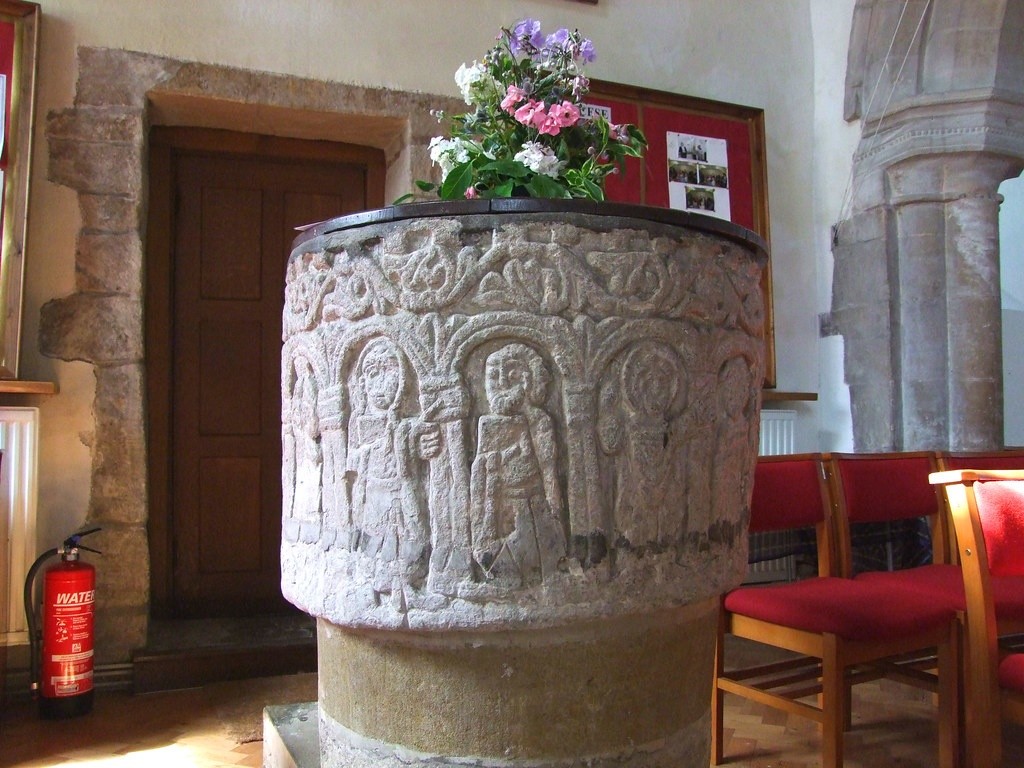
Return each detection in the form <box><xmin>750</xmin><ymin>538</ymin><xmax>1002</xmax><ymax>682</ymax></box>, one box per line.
<box><xmin>389</xmin><ymin>19</ymin><xmax>651</xmax><ymax>205</ymax></box>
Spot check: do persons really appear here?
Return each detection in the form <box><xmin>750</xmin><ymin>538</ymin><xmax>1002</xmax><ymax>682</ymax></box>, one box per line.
<box><xmin>346</xmin><ymin>336</ymin><xmax>443</xmax><ymax>593</ymax></box>
<box><xmin>466</xmin><ymin>344</ymin><xmax>568</xmax><ymax>584</ymax></box>
<box><xmin>670</xmin><ymin>138</ymin><xmax>729</xmax><ymax>214</ymax></box>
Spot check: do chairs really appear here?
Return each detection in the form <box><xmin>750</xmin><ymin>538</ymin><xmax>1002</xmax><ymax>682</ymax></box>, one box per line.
<box><xmin>707</xmin><ymin>447</ymin><xmax>1024</xmax><ymax>768</ymax></box>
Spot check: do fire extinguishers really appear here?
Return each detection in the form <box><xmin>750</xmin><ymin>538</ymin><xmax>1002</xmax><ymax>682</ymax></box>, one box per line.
<box><xmin>17</xmin><ymin>528</ymin><xmax>103</xmax><ymax>721</ymax></box>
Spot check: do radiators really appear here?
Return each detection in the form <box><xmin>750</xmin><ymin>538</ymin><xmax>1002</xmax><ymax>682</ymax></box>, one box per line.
<box><xmin>0</xmin><ymin>406</ymin><xmax>40</xmax><ymax>646</ymax></box>
<box><xmin>742</xmin><ymin>408</ymin><xmax>798</xmax><ymax>584</ymax></box>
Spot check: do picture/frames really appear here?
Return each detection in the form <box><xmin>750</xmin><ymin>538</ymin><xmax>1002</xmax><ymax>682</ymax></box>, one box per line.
<box><xmin>0</xmin><ymin>0</ymin><xmax>42</xmax><ymax>380</ymax></box>
<box><xmin>511</xmin><ymin>62</ymin><xmax>777</xmax><ymax>389</ymax></box>
